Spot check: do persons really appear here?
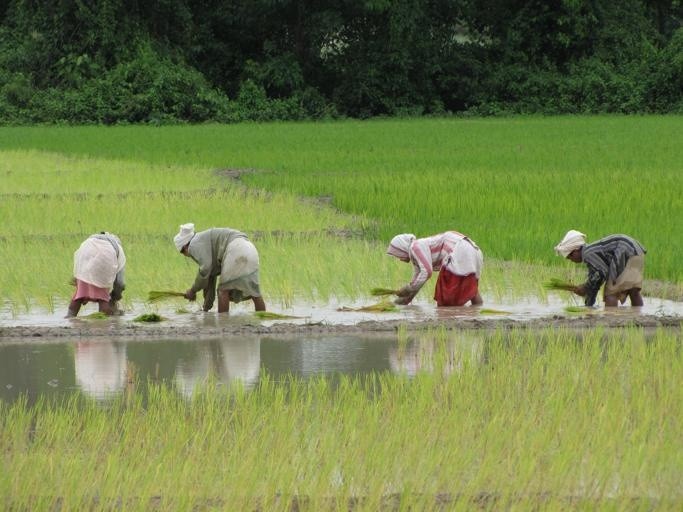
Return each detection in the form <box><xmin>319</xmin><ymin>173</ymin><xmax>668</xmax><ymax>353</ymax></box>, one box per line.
<box><xmin>554</xmin><ymin>229</ymin><xmax>647</xmax><ymax>307</ymax></box>
<box><xmin>66</xmin><ymin>232</ymin><xmax>126</xmax><ymax>318</ymax></box>
<box><xmin>387</xmin><ymin>231</ymin><xmax>484</xmax><ymax>306</ymax></box>
<box><xmin>174</xmin><ymin>223</ymin><xmax>266</xmax><ymax>312</ymax></box>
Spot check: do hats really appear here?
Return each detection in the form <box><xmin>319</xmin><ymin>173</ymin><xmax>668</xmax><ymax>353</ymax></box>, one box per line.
<box><xmin>174</xmin><ymin>223</ymin><xmax>195</xmax><ymax>253</ymax></box>
<box><xmin>554</xmin><ymin>230</ymin><xmax>586</xmax><ymax>259</ymax></box>
